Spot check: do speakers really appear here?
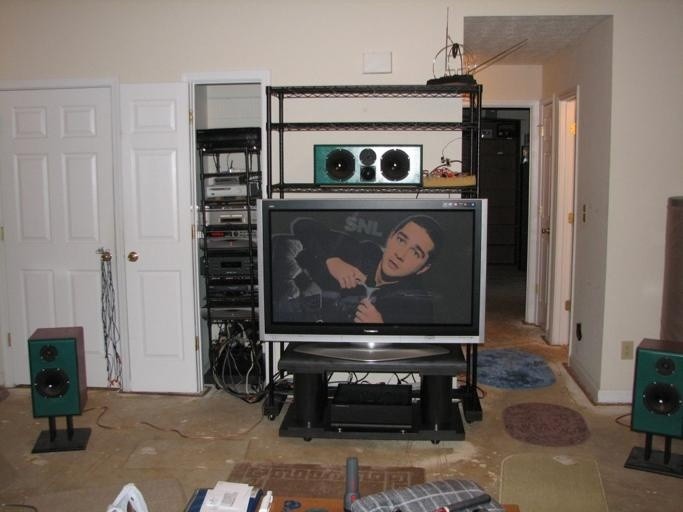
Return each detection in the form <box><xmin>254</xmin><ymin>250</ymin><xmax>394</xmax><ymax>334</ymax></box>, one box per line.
<box><xmin>28</xmin><ymin>326</ymin><xmax>88</xmax><ymax>418</ymax></box>
<box><xmin>630</xmin><ymin>338</ymin><xmax>683</xmax><ymax>440</ymax></box>
<box><xmin>313</xmin><ymin>144</ymin><xmax>423</xmax><ymax>186</ymax></box>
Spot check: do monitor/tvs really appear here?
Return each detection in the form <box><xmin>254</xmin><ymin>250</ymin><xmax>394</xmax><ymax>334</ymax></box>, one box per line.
<box><xmin>256</xmin><ymin>199</ymin><xmax>488</xmax><ymax>361</ymax></box>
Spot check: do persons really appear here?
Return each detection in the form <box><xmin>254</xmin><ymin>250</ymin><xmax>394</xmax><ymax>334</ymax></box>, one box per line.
<box><xmin>292</xmin><ymin>214</ymin><xmax>442</xmax><ymax>325</ymax></box>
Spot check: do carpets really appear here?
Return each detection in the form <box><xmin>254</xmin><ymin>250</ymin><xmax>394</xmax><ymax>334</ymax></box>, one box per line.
<box><xmin>222</xmin><ymin>460</ymin><xmax>424</xmax><ymax>512</ymax></box>
<box><xmin>470</xmin><ymin>347</ymin><xmax>608</xmax><ymax>512</ymax></box>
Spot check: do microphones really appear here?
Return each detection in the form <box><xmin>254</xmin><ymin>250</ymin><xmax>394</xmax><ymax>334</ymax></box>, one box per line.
<box><xmin>343</xmin><ymin>457</ymin><xmax>360</xmax><ymax>512</ymax></box>
<box><xmin>433</xmin><ymin>494</ymin><xmax>491</xmax><ymax>512</ymax></box>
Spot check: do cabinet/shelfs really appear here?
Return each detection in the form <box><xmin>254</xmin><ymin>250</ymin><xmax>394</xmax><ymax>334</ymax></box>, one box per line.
<box><xmin>264</xmin><ymin>85</ymin><xmax>483</xmax><ymax>443</ymax></box>
<box><xmin>196</xmin><ymin>146</ymin><xmax>260</xmax><ymax>383</ymax></box>
